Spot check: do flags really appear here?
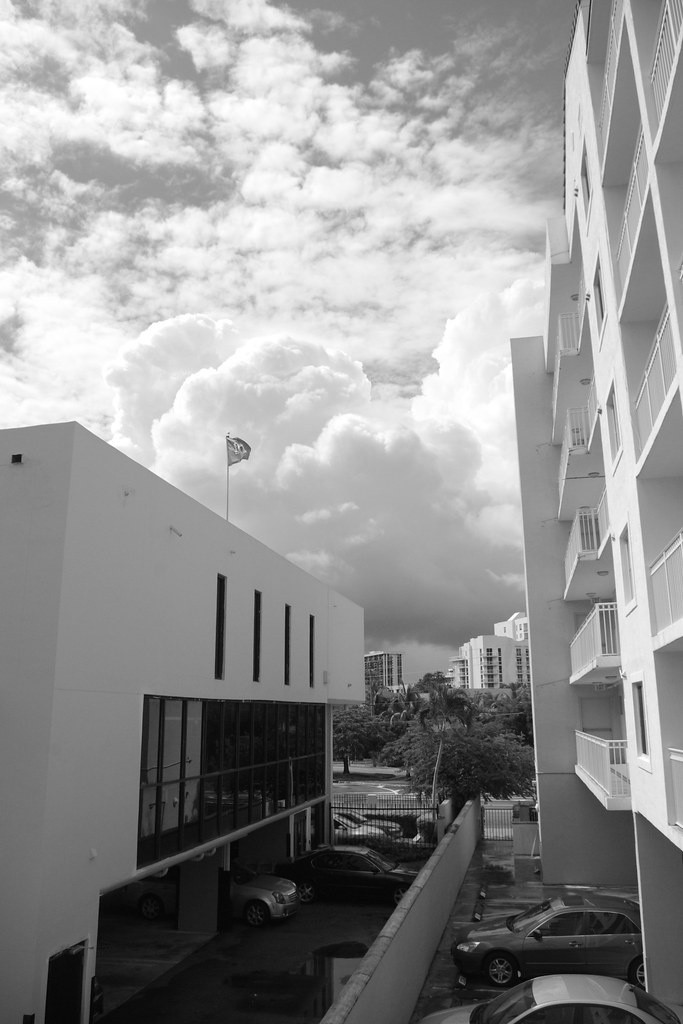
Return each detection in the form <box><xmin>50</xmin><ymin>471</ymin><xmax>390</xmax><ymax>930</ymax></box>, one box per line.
<box><xmin>227</xmin><ymin>437</ymin><xmax>251</xmax><ymax>466</ymax></box>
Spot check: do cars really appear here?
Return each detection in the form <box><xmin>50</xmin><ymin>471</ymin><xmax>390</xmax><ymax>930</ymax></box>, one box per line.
<box><xmin>449</xmin><ymin>889</ymin><xmax>646</xmax><ymax>992</ymax></box>
<box><xmin>273</xmin><ymin>845</ymin><xmax>420</xmax><ymax>909</ymax></box>
<box><xmin>333</xmin><ymin>809</ymin><xmax>403</xmax><ymax>848</ymax></box>
<box><xmin>416</xmin><ymin>975</ymin><xmax>679</xmax><ymax>1024</ymax></box>
<box><xmin>118</xmin><ymin>853</ymin><xmax>302</xmax><ymax>933</ymax></box>
